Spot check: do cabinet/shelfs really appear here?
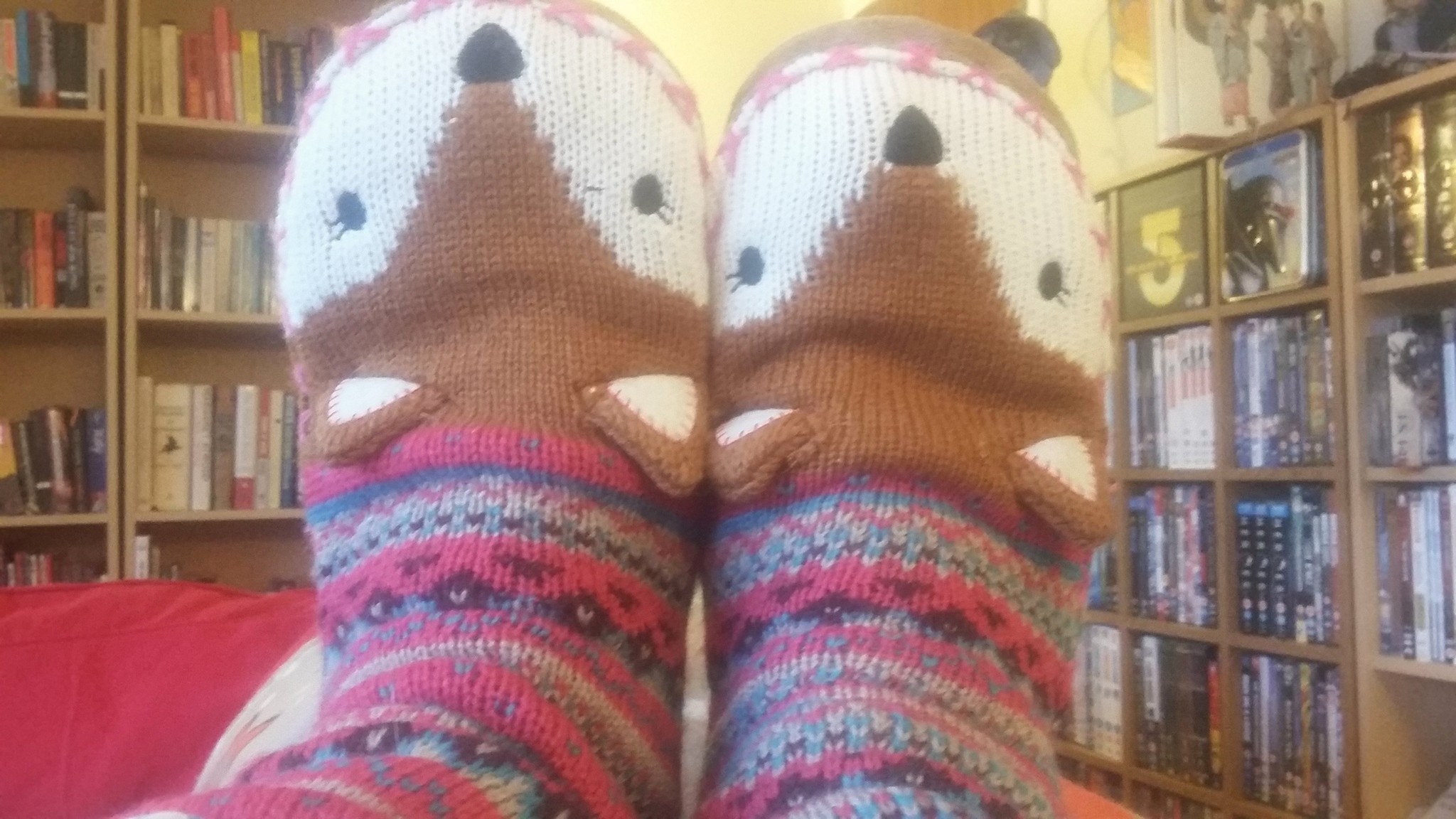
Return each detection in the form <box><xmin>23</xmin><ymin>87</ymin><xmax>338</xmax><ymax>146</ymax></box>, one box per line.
<box><xmin>1054</xmin><ymin>63</ymin><xmax>1456</xmax><ymax>819</ymax></box>
<box><xmin>0</xmin><ymin>0</ymin><xmax>376</xmax><ymax>590</ymax></box>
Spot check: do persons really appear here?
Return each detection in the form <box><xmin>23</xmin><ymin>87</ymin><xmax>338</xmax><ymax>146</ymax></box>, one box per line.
<box><xmin>1207</xmin><ymin>0</ymin><xmax>1420</xmax><ymax>129</ymax></box>
<box><xmin>110</xmin><ymin>0</ymin><xmax>1120</xmax><ymax>819</ymax></box>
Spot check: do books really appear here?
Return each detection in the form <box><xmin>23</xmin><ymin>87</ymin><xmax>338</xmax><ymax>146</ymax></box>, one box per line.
<box><xmin>1072</xmin><ymin>88</ymin><xmax>1456</xmax><ymax>819</ymax></box>
<box><xmin>0</xmin><ymin>541</ymin><xmax>104</xmax><ymax>586</ymax></box>
<box><xmin>140</xmin><ymin>6</ymin><xmax>330</xmax><ymax>126</ymax></box>
<box><xmin>136</xmin><ymin>536</ymin><xmax>310</xmax><ymax>592</ymax></box>
<box><xmin>0</xmin><ymin>184</ymin><xmax>108</xmax><ymax>308</ymax></box>
<box><xmin>0</xmin><ymin>404</ymin><xmax>106</xmax><ymax>515</ymax></box>
<box><xmin>0</xmin><ymin>12</ymin><xmax>101</xmax><ymax>110</ymax></box>
<box><xmin>137</xmin><ymin>375</ymin><xmax>301</xmax><ymax>510</ymax></box>
<box><xmin>136</xmin><ymin>178</ymin><xmax>284</xmax><ymax>316</ymax></box>
